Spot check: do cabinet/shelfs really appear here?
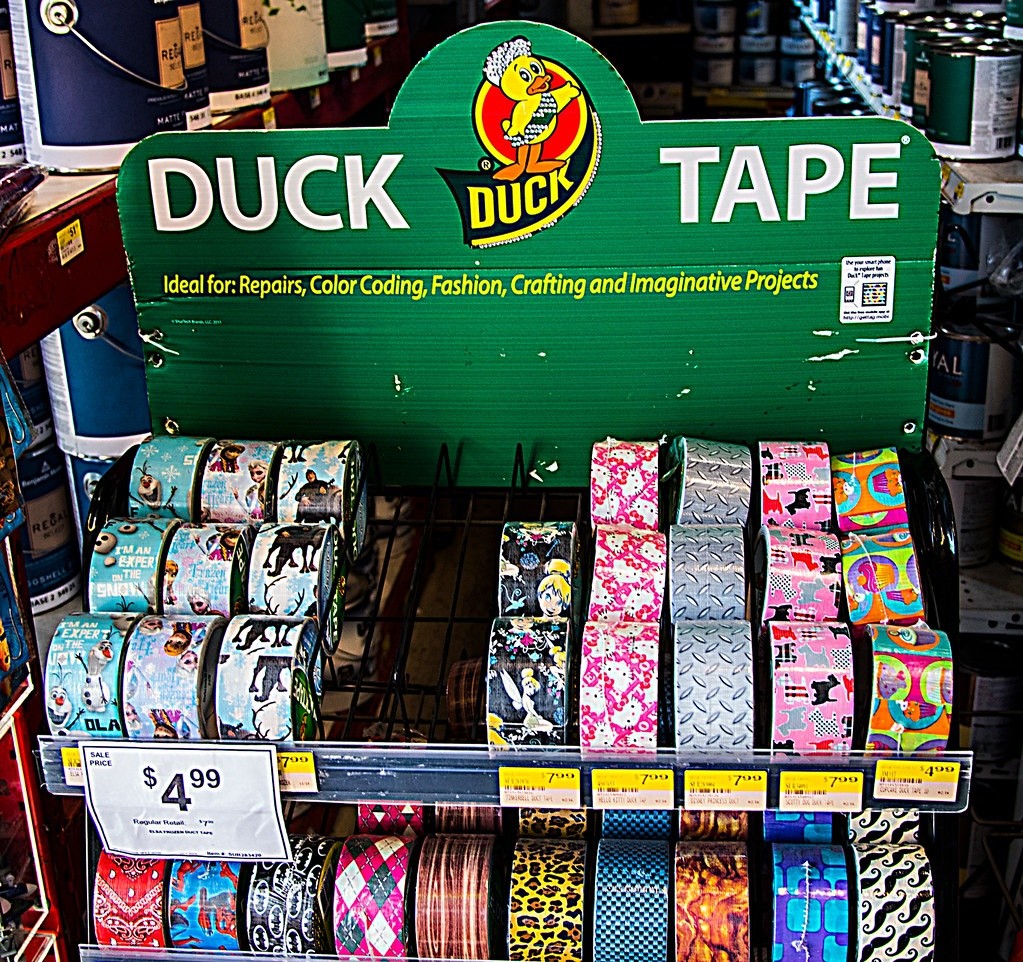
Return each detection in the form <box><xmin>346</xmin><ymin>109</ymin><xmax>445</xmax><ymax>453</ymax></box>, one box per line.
<box><xmin>0</xmin><ymin>17</ymin><xmax>1023</xmax><ymax>962</ymax></box>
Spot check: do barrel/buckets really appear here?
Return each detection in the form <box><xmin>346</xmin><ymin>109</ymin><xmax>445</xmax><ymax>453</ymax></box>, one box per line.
<box><xmin>0</xmin><ymin>0</ymin><xmax>400</xmax><ymax>174</ymax></box>
<box><xmin>926</xmin><ymin>206</ymin><xmax>1023</xmax><ymax>446</ymax></box>
<box><xmin>832</xmin><ymin>0</ymin><xmax>1023</xmax><ymax>164</ymax></box>
<box><xmin>8</xmin><ymin>281</ymin><xmax>150</xmax><ymax>615</ymax></box>
